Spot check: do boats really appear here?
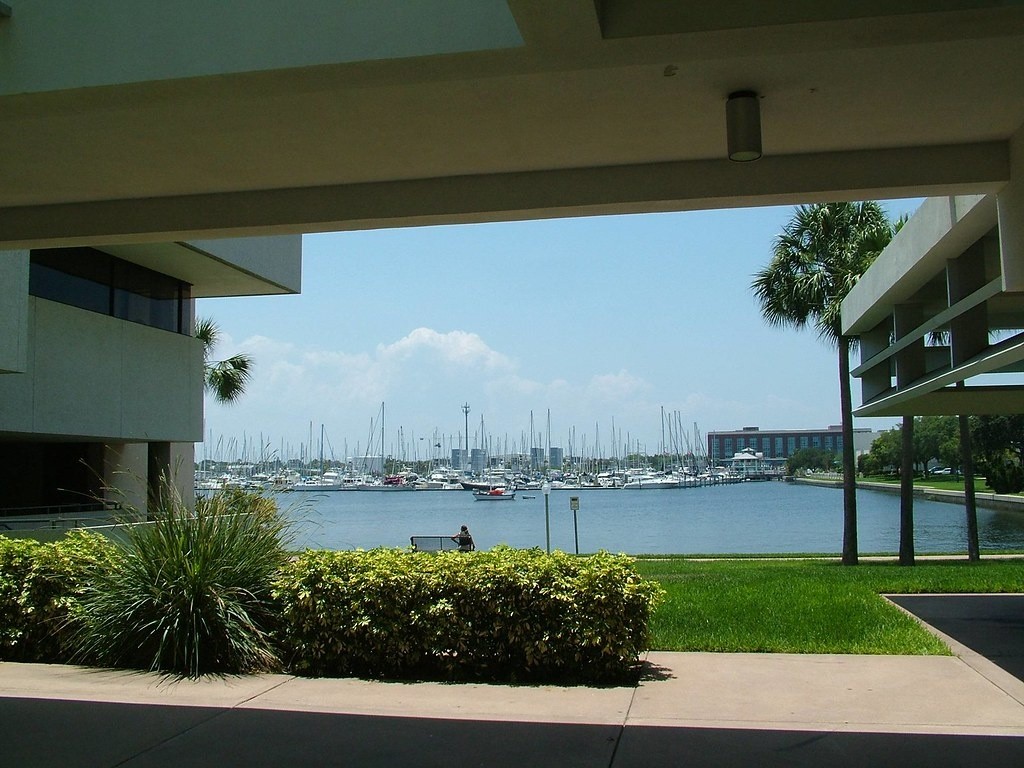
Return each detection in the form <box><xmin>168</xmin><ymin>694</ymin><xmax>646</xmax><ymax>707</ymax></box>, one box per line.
<box><xmin>473</xmin><ymin>488</ymin><xmax>517</xmax><ymax>500</ymax></box>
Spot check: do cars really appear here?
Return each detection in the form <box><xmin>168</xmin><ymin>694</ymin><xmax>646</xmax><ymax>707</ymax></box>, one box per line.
<box><xmin>923</xmin><ymin>466</ymin><xmax>961</xmax><ymax>476</ymax></box>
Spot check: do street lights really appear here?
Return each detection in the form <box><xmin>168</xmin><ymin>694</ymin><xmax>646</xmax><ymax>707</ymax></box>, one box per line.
<box><xmin>541</xmin><ymin>482</ymin><xmax>552</xmax><ymax>555</ymax></box>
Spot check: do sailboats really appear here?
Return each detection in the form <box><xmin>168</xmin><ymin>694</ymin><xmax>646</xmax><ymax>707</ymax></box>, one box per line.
<box><xmin>195</xmin><ymin>400</ymin><xmax>744</xmax><ymax>491</ymax></box>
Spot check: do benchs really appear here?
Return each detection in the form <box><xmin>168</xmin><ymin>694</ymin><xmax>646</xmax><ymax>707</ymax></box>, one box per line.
<box><xmin>410</xmin><ymin>536</ymin><xmax>475</xmax><ymax>553</ymax></box>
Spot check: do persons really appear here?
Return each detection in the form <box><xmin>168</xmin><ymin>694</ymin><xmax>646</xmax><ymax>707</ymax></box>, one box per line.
<box><xmin>451</xmin><ymin>525</ymin><xmax>475</xmax><ymax>553</ymax></box>
